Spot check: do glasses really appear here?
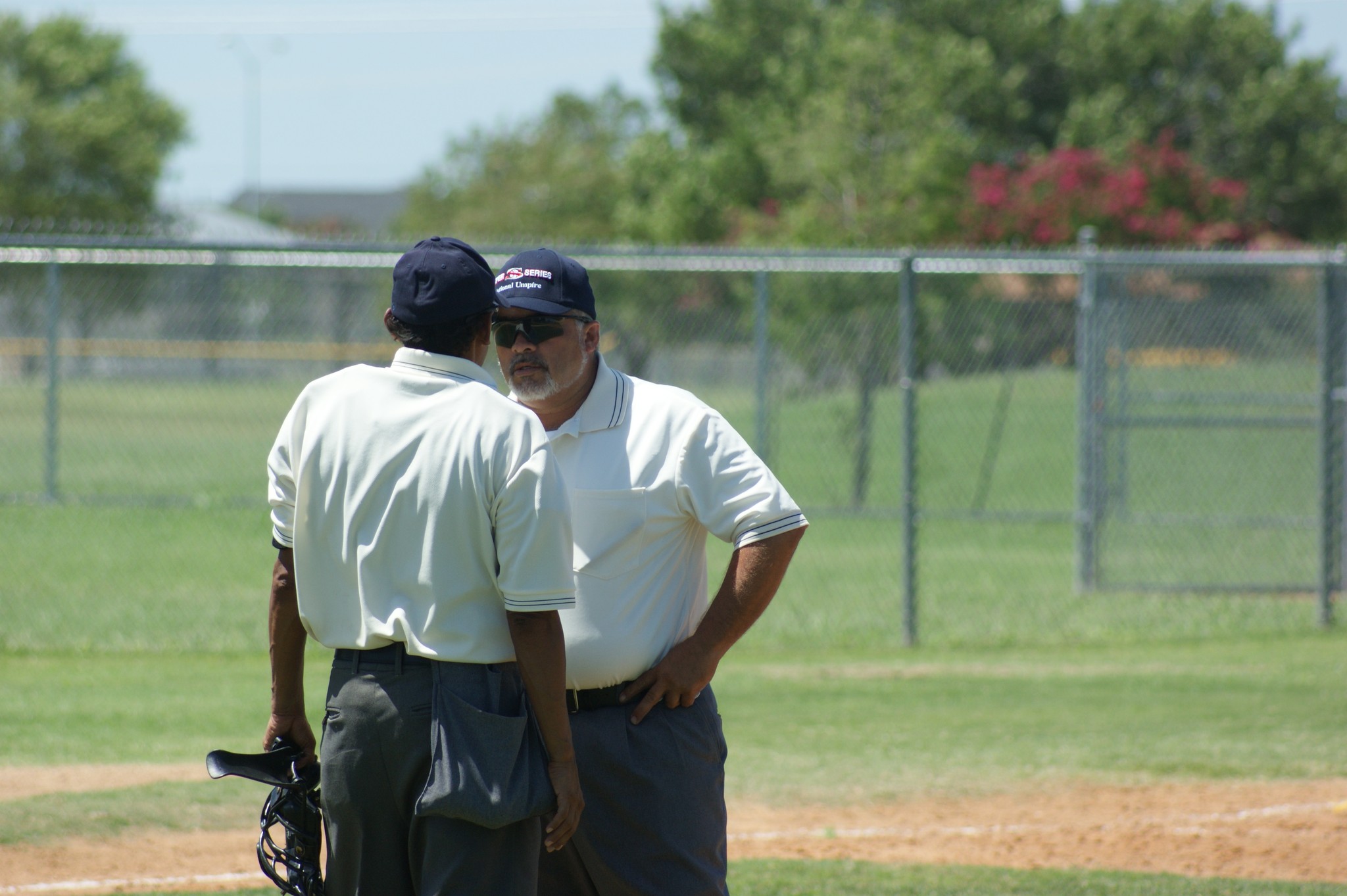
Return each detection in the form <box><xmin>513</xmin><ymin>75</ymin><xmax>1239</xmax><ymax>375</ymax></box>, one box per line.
<box><xmin>485</xmin><ymin>309</ymin><xmax>597</xmax><ymax>348</ymax></box>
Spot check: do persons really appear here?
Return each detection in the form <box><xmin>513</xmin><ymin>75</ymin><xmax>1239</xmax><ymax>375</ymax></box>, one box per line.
<box><xmin>480</xmin><ymin>248</ymin><xmax>806</xmax><ymax>896</ymax></box>
<box><xmin>263</xmin><ymin>236</ymin><xmax>587</xmax><ymax>896</ymax></box>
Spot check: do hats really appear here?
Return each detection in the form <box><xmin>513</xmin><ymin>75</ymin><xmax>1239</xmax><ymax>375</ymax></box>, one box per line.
<box><xmin>391</xmin><ymin>233</ymin><xmax>494</xmax><ymax>328</ymax></box>
<box><xmin>493</xmin><ymin>244</ymin><xmax>598</xmax><ymax>319</ymax></box>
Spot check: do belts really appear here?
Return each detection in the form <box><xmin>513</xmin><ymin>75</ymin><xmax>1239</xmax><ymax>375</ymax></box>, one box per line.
<box><xmin>564</xmin><ymin>679</ymin><xmax>651</xmax><ymax>713</ymax></box>
<box><xmin>333</xmin><ymin>648</ymin><xmax>429</xmax><ymax>666</ymax></box>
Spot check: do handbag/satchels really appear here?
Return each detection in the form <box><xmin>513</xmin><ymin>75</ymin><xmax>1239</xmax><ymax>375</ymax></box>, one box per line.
<box><xmin>411</xmin><ymin>661</ymin><xmax>559</xmax><ymax>830</ymax></box>
